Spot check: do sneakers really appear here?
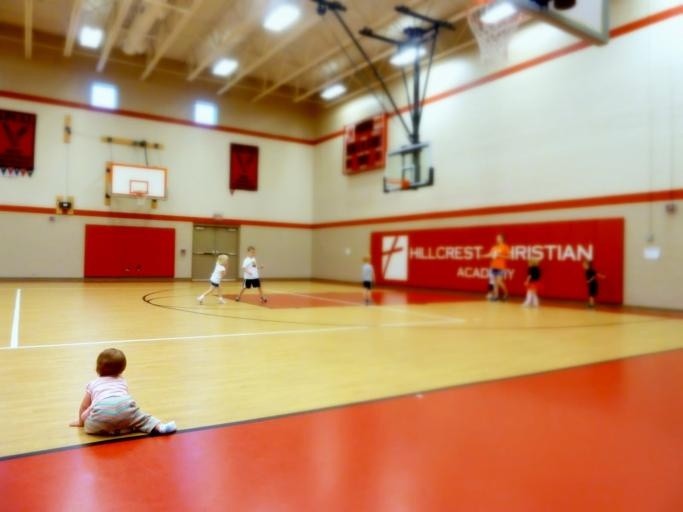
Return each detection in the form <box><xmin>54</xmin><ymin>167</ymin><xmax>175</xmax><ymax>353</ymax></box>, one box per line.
<box><xmin>197</xmin><ymin>296</ymin><xmax>268</xmax><ymax>304</ymax></box>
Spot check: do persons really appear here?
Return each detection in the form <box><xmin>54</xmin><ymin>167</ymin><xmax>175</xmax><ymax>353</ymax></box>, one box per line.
<box><xmin>359</xmin><ymin>256</ymin><xmax>375</xmax><ymax>306</ymax></box>
<box><xmin>236</xmin><ymin>246</ymin><xmax>267</xmax><ymax>303</ymax></box>
<box><xmin>198</xmin><ymin>254</ymin><xmax>229</xmax><ymax>304</ymax></box>
<box><xmin>69</xmin><ymin>348</ymin><xmax>176</xmax><ymax>434</ymax></box>
<box><xmin>522</xmin><ymin>257</ymin><xmax>541</xmax><ymax>308</ymax></box>
<box><xmin>478</xmin><ymin>233</ymin><xmax>508</xmax><ymax>300</ymax></box>
<box><xmin>583</xmin><ymin>259</ymin><xmax>606</xmax><ymax>307</ymax></box>
